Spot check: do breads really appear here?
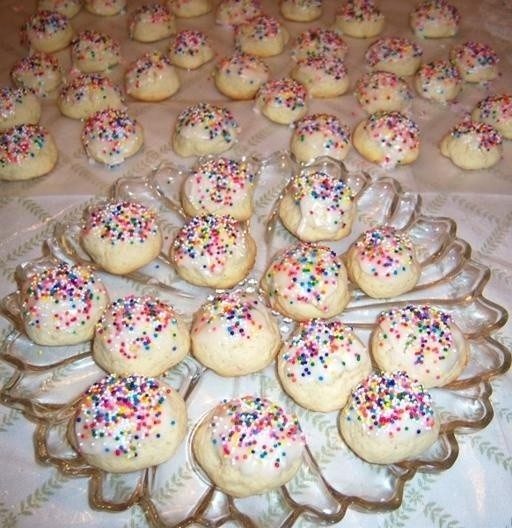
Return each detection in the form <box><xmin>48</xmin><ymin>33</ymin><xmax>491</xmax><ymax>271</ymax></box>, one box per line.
<box><xmin>22</xmin><ymin>156</ymin><xmax>469</xmax><ymax>496</ymax></box>
<box><xmin>1</xmin><ymin>0</ymin><xmax>510</xmax><ymax>180</ymax></box>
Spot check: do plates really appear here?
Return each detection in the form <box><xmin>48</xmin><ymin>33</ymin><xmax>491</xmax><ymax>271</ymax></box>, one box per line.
<box><xmin>1</xmin><ymin>147</ymin><xmax>511</xmax><ymax>528</ymax></box>
